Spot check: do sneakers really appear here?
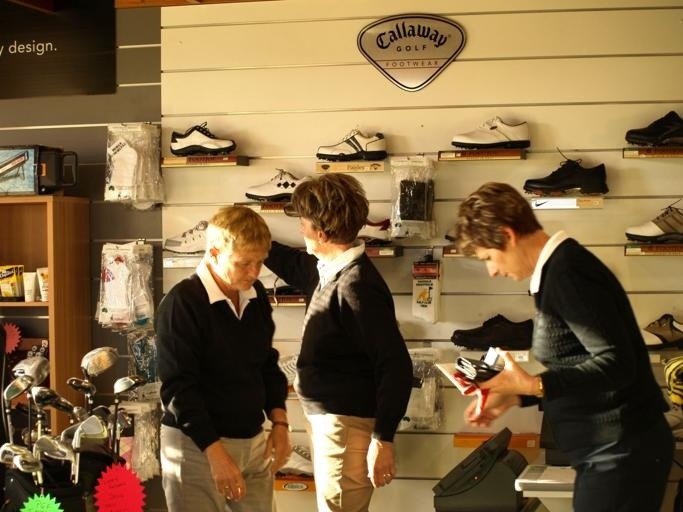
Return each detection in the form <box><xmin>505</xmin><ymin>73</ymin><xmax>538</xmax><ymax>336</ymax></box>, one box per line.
<box><xmin>279</xmin><ymin>444</ymin><xmax>314</xmax><ymax>477</ymax></box>
<box><xmin>451</xmin><ymin>115</ymin><xmax>532</xmax><ymax>151</ymax></box>
<box><xmin>278</xmin><ymin>353</ymin><xmax>298</xmax><ymax>385</ymax></box>
<box><xmin>625</xmin><ymin>199</ymin><xmax>683</xmax><ymax>244</ymax></box>
<box><xmin>164</xmin><ymin>220</ymin><xmax>209</xmax><ymax>254</ymax></box>
<box><xmin>450</xmin><ymin>310</ymin><xmax>534</xmax><ymax>351</ymax></box>
<box><xmin>624</xmin><ymin>110</ymin><xmax>683</xmax><ymax>148</ymax></box>
<box><xmin>355</xmin><ymin>218</ymin><xmax>395</xmax><ymax>248</ymax></box>
<box><xmin>164</xmin><ymin>111</ymin><xmax>239</xmax><ymax>158</ymax></box>
<box><xmin>522</xmin><ymin>158</ymin><xmax>611</xmax><ymax>197</ymax></box>
<box><xmin>317</xmin><ymin>128</ymin><xmax>389</xmax><ymax>161</ymax></box>
<box><xmin>245</xmin><ymin>167</ymin><xmax>313</xmax><ymax>202</ymax></box>
<box><xmin>640</xmin><ymin>313</ymin><xmax>683</xmax><ymax>351</ymax></box>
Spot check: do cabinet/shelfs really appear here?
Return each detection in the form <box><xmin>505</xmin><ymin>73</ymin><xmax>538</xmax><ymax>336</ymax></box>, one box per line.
<box><xmin>1</xmin><ymin>195</ymin><xmax>88</xmax><ymax>435</ymax></box>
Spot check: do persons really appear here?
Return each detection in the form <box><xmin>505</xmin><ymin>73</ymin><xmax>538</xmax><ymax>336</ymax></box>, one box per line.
<box><xmin>453</xmin><ymin>181</ymin><xmax>677</xmax><ymax>512</ymax></box>
<box><xmin>153</xmin><ymin>205</ymin><xmax>295</xmax><ymax>511</ymax></box>
<box><xmin>263</xmin><ymin>172</ymin><xmax>413</xmax><ymax>512</ymax></box>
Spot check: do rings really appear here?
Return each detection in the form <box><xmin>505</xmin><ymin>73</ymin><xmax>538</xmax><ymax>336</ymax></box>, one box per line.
<box><xmin>224</xmin><ymin>486</ymin><xmax>229</xmax><ymax>489</ymax></box>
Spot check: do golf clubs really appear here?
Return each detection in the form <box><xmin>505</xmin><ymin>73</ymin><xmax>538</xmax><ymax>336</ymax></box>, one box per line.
<box><xmin>0</xmin><ymin>346</ymin><xmax>144</xmax><ymax>497</ymax></box>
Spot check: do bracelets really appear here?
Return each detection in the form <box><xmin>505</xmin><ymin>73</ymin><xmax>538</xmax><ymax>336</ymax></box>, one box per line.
<box><xmin>269</xmin><ymin>421</ymin><xmax>292</xmax><ymax>432</ymax></box>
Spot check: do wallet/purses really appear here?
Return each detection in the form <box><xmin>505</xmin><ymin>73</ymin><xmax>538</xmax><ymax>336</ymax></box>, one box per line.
<box><xmin>456</xmin><ymin>356</ymin><xmax>500</xmax><ymax>378</ymax></box>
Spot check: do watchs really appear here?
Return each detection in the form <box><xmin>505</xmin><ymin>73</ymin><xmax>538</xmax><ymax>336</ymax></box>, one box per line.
<box><xmin>533</xmin><ymin>374</ymin><xmax>545</xmax><ymax>399</ymax></box>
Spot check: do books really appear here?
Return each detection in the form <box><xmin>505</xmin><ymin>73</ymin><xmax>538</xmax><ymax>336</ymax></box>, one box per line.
<box><xmin>434</xmin><ymin>353</ymin><xmax>548</xmax><ymax>394</ymax></box>
